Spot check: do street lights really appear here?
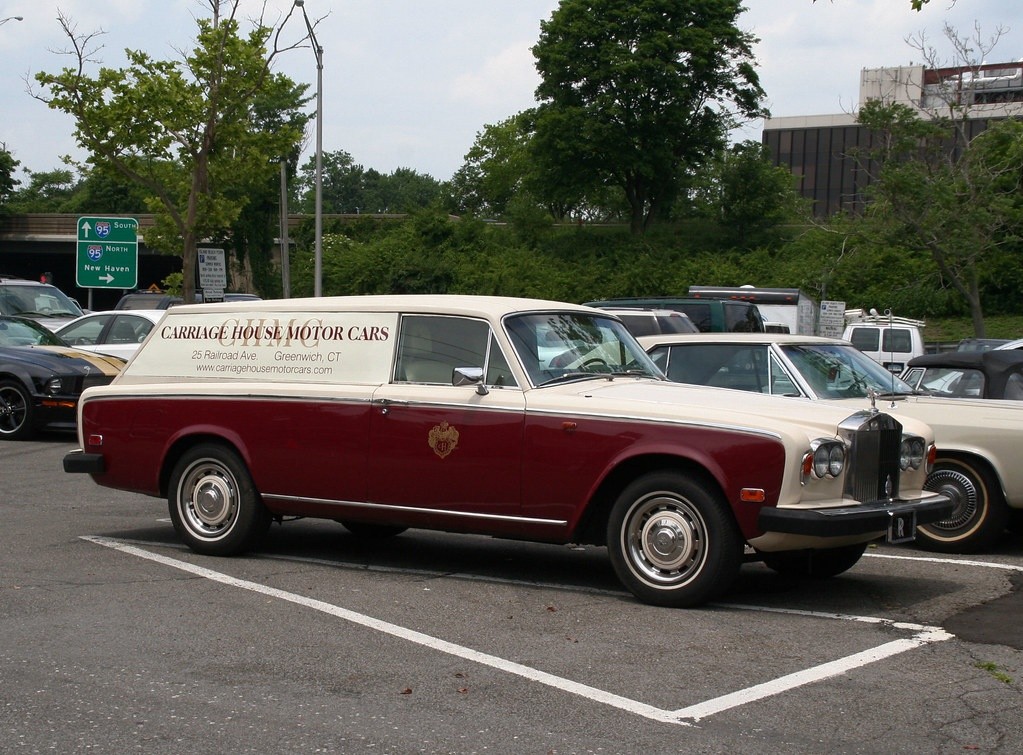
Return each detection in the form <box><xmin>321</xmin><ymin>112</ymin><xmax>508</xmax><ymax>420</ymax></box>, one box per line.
<box><xmin>294</xmin><ymin>0</ymin><xmax>325</xmax><ymax>297</ymax></box>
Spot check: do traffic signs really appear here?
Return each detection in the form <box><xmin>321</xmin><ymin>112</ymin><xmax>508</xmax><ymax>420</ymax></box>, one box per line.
<box><xmin>75</xmin><ymin>215</ymin><xmax>139</xmax><ymax>290</ymax></box>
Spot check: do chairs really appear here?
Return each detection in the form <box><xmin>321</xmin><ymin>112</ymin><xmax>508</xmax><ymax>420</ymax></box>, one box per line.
<box><xmin>113</xmin><ymin>323</ymin><xmax>139</xmax><ymax>343</ymax></box>
<box><xmin>403</xmin><ymin>358</ymin><xmax>454</xmax><ymax>385</ymax></box>
<box><xmin>487</xmin><ymin>359</ymin><xmax>516</xmax><ymax>386</ymax></box>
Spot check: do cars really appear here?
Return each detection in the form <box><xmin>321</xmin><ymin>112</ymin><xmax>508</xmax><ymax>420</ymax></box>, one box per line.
<box><xmin>533</xmin><ymin>281</ymin><xmax>1023</xmax><ymax>555</ymax></box>
<box><xmin>0</xmin><ymin>276</ymin><xmax>262</xmax><ymax>442</ymax></box>
<box><xmin>61</xmin><ymin>292</ymin><xmax>957</xmax><ymax>609</ymax></box>
